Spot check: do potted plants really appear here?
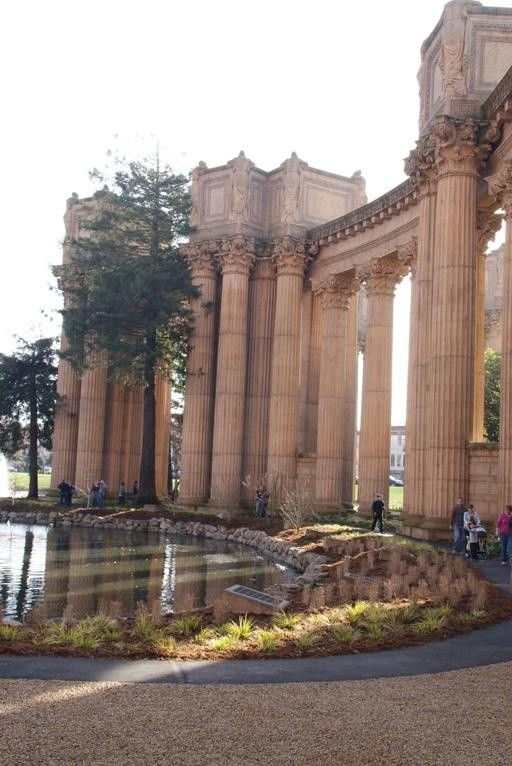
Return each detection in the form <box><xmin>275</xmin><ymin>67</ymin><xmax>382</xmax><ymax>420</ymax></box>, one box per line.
<box><xmin>132</xmin><ymin>488</ymin><xmax>163</xmax><ymax>512</ymax></box>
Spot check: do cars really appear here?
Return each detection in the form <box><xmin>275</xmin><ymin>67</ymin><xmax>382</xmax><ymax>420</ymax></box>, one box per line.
<box><xmin>7</xmin><ymin>466</ymin><xmax>17</xmax><ymax>473</ymax></box>
<box><xmin>43</xmin><ymin>466</ymin><xmax>52</xmax><ymax>474</ymax></box>
<box><xmin>389</xmin><ymin>473</ymin><xmax>404</xmax><ymax>487</ymax></box>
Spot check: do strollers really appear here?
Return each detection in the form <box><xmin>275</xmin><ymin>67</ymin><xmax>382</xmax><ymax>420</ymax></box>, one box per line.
<box><xmin>465</xmin><ymin>524</ymin><xmax>489</xmax><ymax>561</ymax></box>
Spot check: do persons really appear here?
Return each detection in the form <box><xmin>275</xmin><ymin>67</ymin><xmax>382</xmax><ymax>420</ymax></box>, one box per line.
<box><xmin>132</xmin><ymin>480</ymin><xmax>138</xmax><ymax>507</ymax></box>
<box><xmin>57</xmin><ymin>480</ymin><xmax>76</xmax><ymax>511</ymax></box>
<box><xmin>370</xmin><ymin>494</ymin><xmax>385</xmax><ymax>534</ymax></box>
<box><xmin>255</xmin><ymin>491</ymin><xmax>267</xmax><ymax>518</ymax></box>
<box><xmin>448</xmin><ymin>497</ymin><xmax>483</xmax><ymax>560</ymax></box>
<box><xmin>117</xmin><ymin>482</ymin><xmax>126</xmax><ymax>506</ymax></box>
<box><xmin>90</xmin><ymin>482</ymin><xmax>107</xmax><ymax>509</ymax></box>
<box><xmin>495</xmin><ymin>504</ymin><xmax>511</xmax><ymax>566</ymax></box>
